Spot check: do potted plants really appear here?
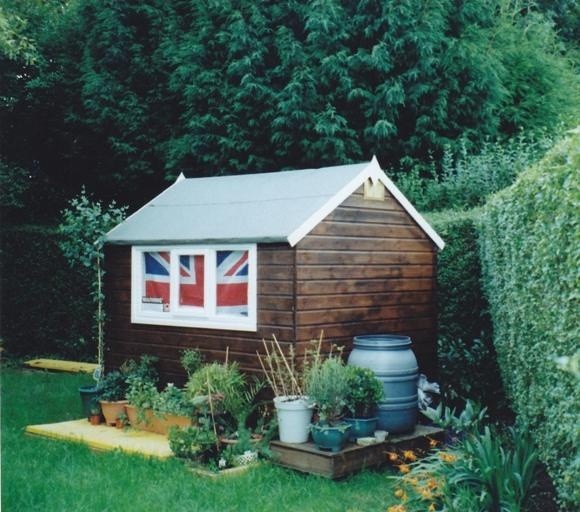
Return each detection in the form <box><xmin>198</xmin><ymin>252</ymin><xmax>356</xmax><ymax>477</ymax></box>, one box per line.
<box><xmin>89</xmin><ymin>329</ymin><xmax>382</xmax><ymax>469</ymax></box>
<box><xmin>54</xmin><ymin>182</ymin><xmax>127</xmax><ymax>420</ymax></box>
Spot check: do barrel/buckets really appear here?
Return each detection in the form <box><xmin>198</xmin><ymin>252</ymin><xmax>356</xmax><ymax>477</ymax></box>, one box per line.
<box><xmin>348</xmin><ymin>334</ymin><xmax>419</xmax><ymax>433</ymax></box>
<box><xmin>273</xmin><ymin>394</ymin><xmax>313</xmax><ymax>444</ymax></box>
